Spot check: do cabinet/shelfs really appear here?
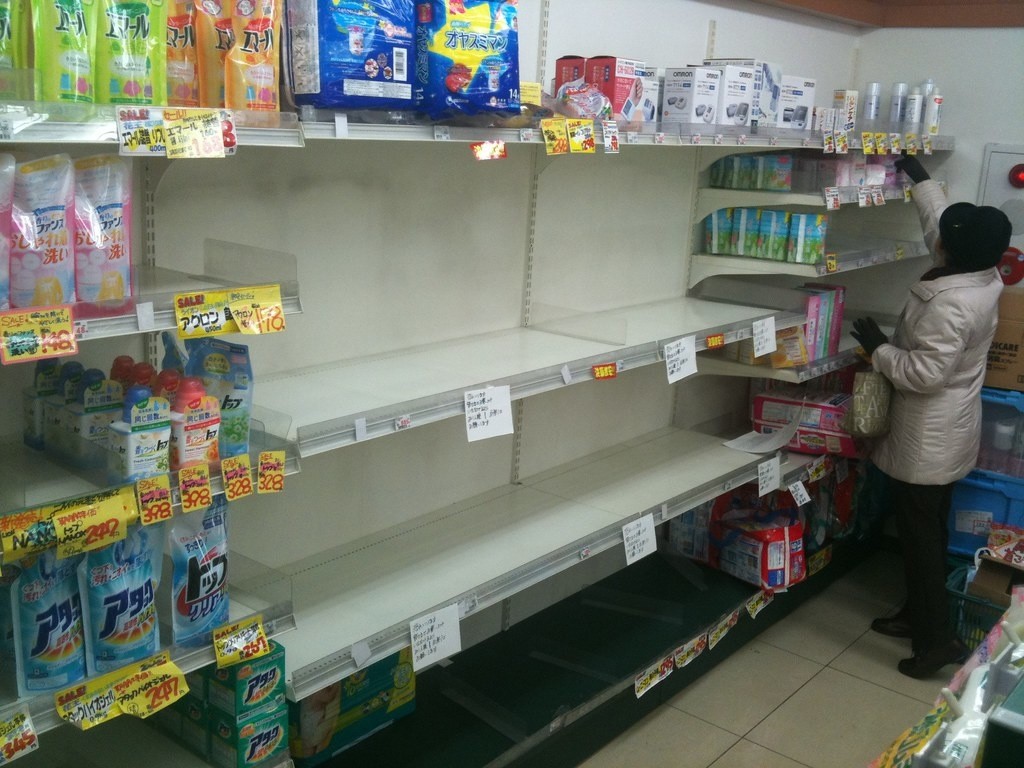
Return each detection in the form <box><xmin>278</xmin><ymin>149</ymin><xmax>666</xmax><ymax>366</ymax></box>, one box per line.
<box><xmin>1</xmin><ymin>105</ymin><xmax>306</xmax><ymax>768</ymax></box>
<box><xmin>148</xmin><ymin>109</ymin><xmax>682</xmax><ymax>768</ymax></box>
<box><xmin>509</xmin><ymin>130</ymin><xmax>894</xmax><ymax>683</ymax></box>
<box><xmin>673</xmin><ymin>130</ymin><xmax>959</xmax><ymax>595</ymax></box>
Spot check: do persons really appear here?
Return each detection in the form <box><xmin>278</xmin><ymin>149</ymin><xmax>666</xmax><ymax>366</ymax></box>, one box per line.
<box><xmin>850</xmin><ymin>150</ymin><xmax>1013</xmax><ymax>679</ymax></box>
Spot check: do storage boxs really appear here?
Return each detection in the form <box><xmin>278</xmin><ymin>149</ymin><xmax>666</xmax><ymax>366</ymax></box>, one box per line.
<box><xmin>983</xmin><ymin>285</ymin><xmax>1024</xmax><ymax>391</ymax></box>
<box><xmin>705</xmin><ymin>208</ymin><xmax>845</xmax><ymax>366</ymax></box>
<box><xmin>946</xmin><ymin>468</ymin><xmax>1024</xmax><ymax>555</ymax></box>
<box><xmin>963</xmin><ymin>553</ymin><xmax>1023</xmax><ymax>632</ymax></box>
<box><xmin>941</xmin><ymin>567</ymin><xmax>1006</xmax><ymax>663</ymax></box>
<box><xmin>975</xmin><ymin>387</ymin><xmax>1024</xmax><ymax>481</ymax></box>
<box><xmin>551</xmin><ymin>56</ymin><xmax>857</xmax><ymax>135</ymax></box>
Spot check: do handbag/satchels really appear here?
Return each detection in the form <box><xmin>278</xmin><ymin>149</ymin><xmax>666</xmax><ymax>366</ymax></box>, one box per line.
<box><xmin>838</xmin><ymin>369</ymin><xmax>891</xmax><ymax>438</ymax></box>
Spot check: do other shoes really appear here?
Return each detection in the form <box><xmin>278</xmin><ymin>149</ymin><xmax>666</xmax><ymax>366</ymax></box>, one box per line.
<box><xmin>898</xmin><ymin>636</ymin><xmax>965</xmax><ymax>680</ymax></box>
<box><xmin>871</xmin><ymin>615</ymin><xmax>914</xmax><ymax>638</ymax></box>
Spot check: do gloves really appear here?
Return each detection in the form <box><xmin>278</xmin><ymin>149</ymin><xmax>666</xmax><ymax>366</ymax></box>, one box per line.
<box><xmin>894</xmin><ymin>149</ymin><xmax>931</xmax><ymax>184</ymax></box>
<box><xmin>850</xmin><ymin>317</ymin><xmax>889</xmax><ymax>358</ymax></box>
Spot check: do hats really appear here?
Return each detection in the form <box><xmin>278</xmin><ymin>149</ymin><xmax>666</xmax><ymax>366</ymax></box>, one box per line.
<box><xmin>940</xmin><ymin>203</ymin><xmax>1012</xmax><ymax>273</ymax></box>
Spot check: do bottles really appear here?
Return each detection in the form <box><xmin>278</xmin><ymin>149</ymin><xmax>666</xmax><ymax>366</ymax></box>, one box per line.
<box><xmin>902</xmin><ymin>87</ymin><xmax>923</xmax><ymax>134</ymax></box>
<box><xmin>888</xmin><ymin>82</ymin><xmax>909</xmax><ymax>133</ymax></box>
<box><xmin>920</xmin><ymin>78</ymin><xmax>936</xmax><ymax>132</ymax></box>
<box><xmin>860</xmin><ymin>82</ymin><xmax>882</xmax><ymax>131</ymax></box>
<box><xmin>923</xmin><ymin>87</ymin><xmax>944</xmax><ymax>135</ymax></box>
